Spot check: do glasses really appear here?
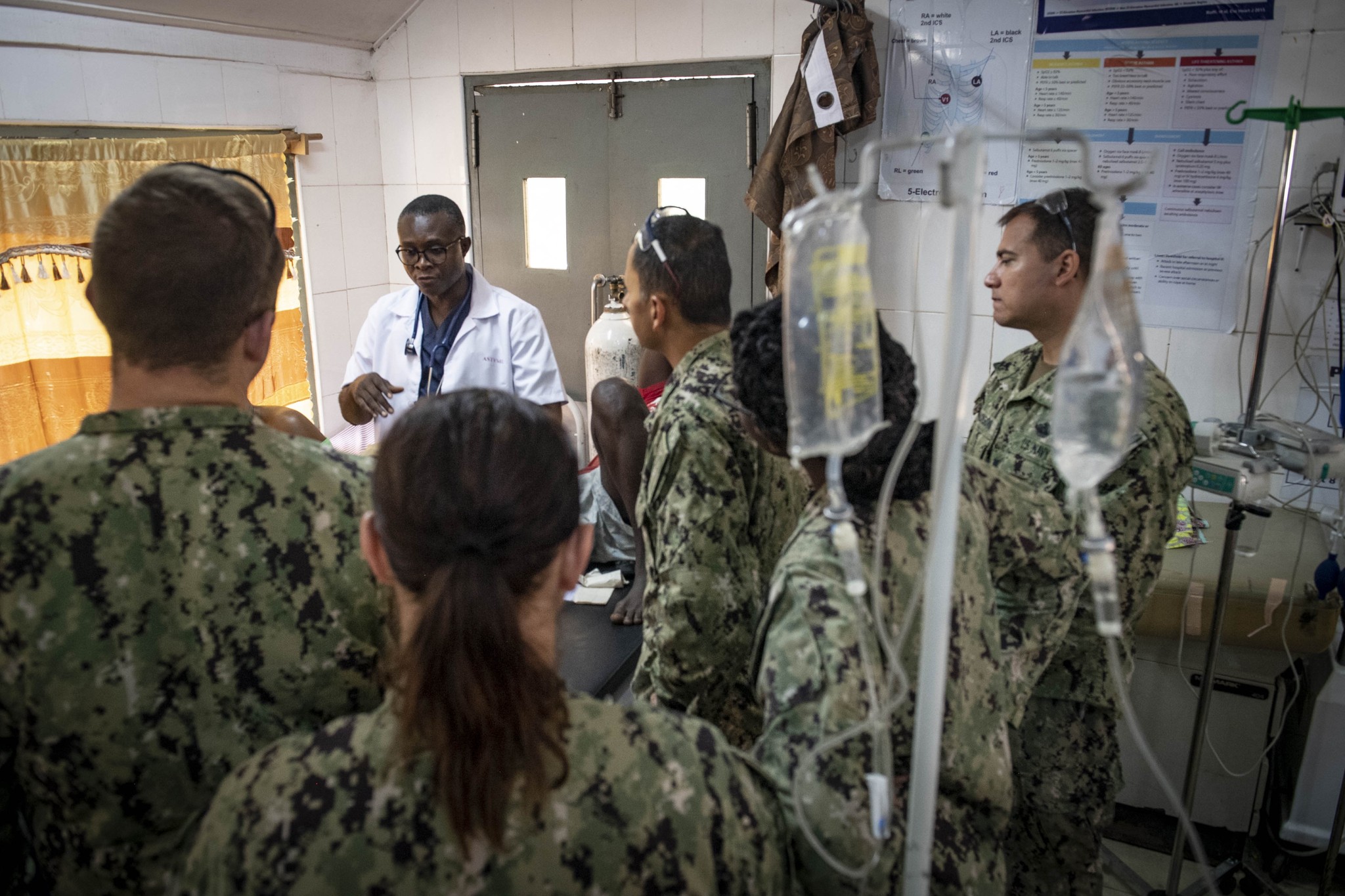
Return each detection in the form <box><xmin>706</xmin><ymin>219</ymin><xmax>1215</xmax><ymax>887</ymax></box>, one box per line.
<box><xmin>170</xmin><ymin>159</ymin><xmax>279</xmax><ymax>323</ymax></box>
<box><xmin>395</xmin><ymin>236</ymin><xmax>464</xmax><ymax>266</ymax></box>
<box><xmin>1041</xmin><ymin>185</ymin><xmax>1081</xmax><ymax>281</ymax></box>
<box><xmin>709</xmin><ymin>370</ymin><xmax>757</xmax><ymax>419</ymax></box>
<box><xmin>635</xmin><ymin>205</ymin><xmax>691</xmax><ymax>294</ymax></box>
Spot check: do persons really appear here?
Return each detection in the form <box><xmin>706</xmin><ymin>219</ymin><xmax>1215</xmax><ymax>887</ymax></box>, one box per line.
<box><xmin>560</xmin><ymin>340</ymin><xmax>674</xmax><ymax>620</ymax></box>
<box><xmin>620</xmin><ymin>214</ymin><xmax>806</xmax><ymax>758</ymax></box>
<box><xmin>0</xmin><ymin>163</ymin><xmax>402</xmax><ymax>896</ymax></box>
<box><xmin>250</xmin><ymin>401</ymin><xmax>334</xmax><ymax>449</ymax></box>
<box><xmin>962</xmin><ymin>186</ymin><xmax>1196</xmax><ymax>895</ymax></box>
<box><xmin>336</xmin><ymin>192</ymin><xmax>568</xmax><ymax>462</ymax></box>
<box><xmin>725</xmin><ymin>290</ymin><xmax>1017</xmax><ymax>896</ymax></box>
<box><xmin>177</xmin><ymin>387</ymin><xmax>807</xmax><ymax>896</ymax></box>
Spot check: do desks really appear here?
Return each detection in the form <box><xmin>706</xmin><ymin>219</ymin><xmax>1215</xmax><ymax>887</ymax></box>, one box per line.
<box><xmin>553</xmin><ymin>553</ymin><xmax>649</xmax><ymax>703</ymax></box>
<box><xmin>1131</xmin><ymin>496</ymin><xmax>1345</xmax><ymax>896</ymax></box>
